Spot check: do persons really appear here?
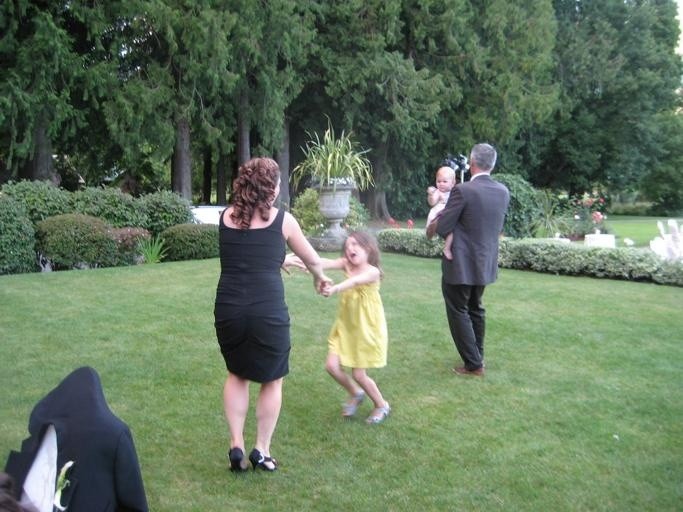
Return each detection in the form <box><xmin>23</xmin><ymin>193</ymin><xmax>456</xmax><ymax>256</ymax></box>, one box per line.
<box><xmin>424</xmin><ymin>164</ymin><xmax>459</xmax><ymax>261</ymax></box>
<box><xmin>433</xmin><ymin>140</ymin><xmax>512</xmax><ymax>379</ymax></box>
<box><xmin>212</xmin><ymin>156</ymin><xmax>334</xmax><ymax>474</ymax></box>
<box><xmin>298</xmin><ymin>229</ymin><xmax>392</xmax><ymax>427</ymax></box>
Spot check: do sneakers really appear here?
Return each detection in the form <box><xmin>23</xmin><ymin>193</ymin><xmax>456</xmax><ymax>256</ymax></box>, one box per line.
<box><xmin>453</xmin><ymin>362</ymin><xmax>485</xmax><ymax>379</ymax></box>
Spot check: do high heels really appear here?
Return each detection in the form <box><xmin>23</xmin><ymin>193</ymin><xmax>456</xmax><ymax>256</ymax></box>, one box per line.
<box><xmin>249</xmin><ymin>448</ymin><xmax>279</xmax><ymax>472</ymax></box>
<box><xmin>228</xmin><ymin>447</ymin><xmax>249</xmax><ymax>473</ymax></box>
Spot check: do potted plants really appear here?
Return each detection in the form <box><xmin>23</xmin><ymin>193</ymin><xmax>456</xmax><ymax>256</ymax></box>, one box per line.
<box><xmin>287</xmin><ymin>107</ymin><xmax>378</xmax><ymax>251</ymax></box>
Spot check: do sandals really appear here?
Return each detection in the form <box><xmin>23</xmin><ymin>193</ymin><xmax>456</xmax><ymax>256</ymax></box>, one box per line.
<box><xmin>365</xmin><ymin>401</ymin><xmax>392</xmax><ymax>425</ymax></box>
<box><xmin>340</xmin><ymin>388</ymin><xmax>365</xmax><ymax>418</ymax></box>
